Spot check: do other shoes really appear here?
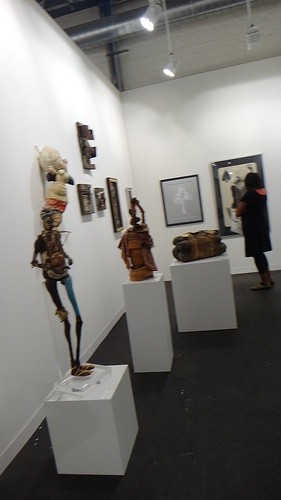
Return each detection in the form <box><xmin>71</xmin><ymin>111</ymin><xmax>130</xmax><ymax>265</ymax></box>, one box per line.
<box><xmin>249</xmin><ymin>280</ymin><xmax>272</xmax><ymax>290</ymax></box>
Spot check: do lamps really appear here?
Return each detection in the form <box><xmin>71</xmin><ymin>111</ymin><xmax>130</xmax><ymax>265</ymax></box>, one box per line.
<box><xmin>140</xmin><ymin>0</ymin><xmax>162</xmax><ymax>32</ymax></box>
<box><xmin>163</xmin><ymin>53</ymin><xmax>178</xmax><ymax>77</ymax></box>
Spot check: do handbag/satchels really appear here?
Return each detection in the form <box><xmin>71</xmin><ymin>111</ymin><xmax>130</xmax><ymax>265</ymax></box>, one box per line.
<box><xmin>227</xmin><ymin>207</ymin><xmax>243</xmax><ymax>235</ymax></box>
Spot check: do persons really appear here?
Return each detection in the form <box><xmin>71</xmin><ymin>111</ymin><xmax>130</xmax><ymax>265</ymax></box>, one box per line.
<box><xmin>235</xmin><ymin>172</ymin><xmax>275</xmax><ymax>291</ymax></box>
<box><xmin>29</xmin><ymin>206</ymin><xmax>95</xmax><ymax>376</ymax></box>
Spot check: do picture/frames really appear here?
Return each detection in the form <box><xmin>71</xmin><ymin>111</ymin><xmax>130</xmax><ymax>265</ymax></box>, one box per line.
<box><xmin>160</xmin><ymin>174</ymin><xmax>204</xmax><ymax>227</ymax></box>
<box><xmin>211</xmin><ymin>154</ymin><xmax>266</xmax><ymax>236</ymax></box>
<box><xmin>107</xmin><ymin>177</ymin><xmax>124</xmax><ymax>233</ymax></box>
<box><xmin>125</xmin><ymin>187</ymin><xmax>132</xmax><ymax>209</ymax></box>
<box><xmin>77</xmin><ymin>184</ymin><xmax>95</xmax><ymax>216</ymax></box>
